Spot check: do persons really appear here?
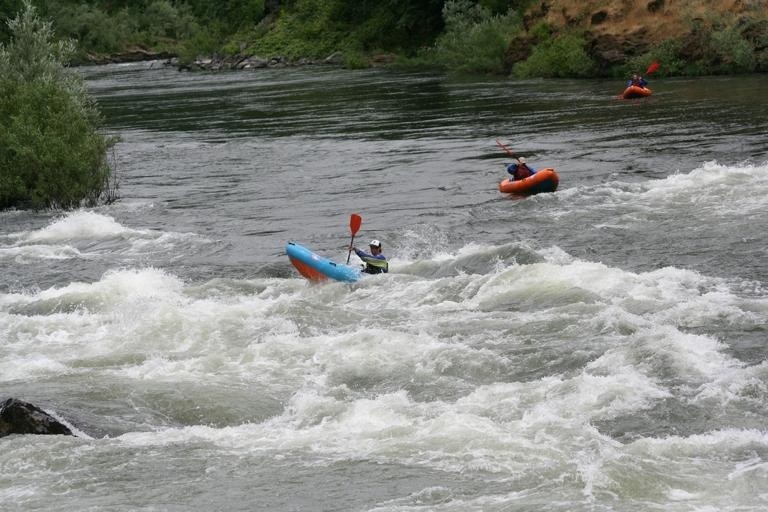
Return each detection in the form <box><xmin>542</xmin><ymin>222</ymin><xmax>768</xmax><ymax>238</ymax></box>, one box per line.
<box><xmin>506</xmin><ymin>156</ymin><xmax>537</xmax><ymax>182</ymax></box>
<box><xmin>348</xmin><ymin>240</ymin><xmax>389</xmax><ymax>275</ymax></box>
<box><xmin>627</xmin><ymin>73</ymin><xmax>649</xmax><ymax>89</ymax></box>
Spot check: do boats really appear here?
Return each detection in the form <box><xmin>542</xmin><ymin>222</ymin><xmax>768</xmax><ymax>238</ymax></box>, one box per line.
<box><xmin>622</xmin><ymin>85</ymin><xmax>651</xmax><ymax>99</ymax></box>
<box><xmin>286</xmin><ymin>241</ymin><xmax>358</xmax><ymax>284</ymax></box>
<box><xmin>500</xmin><ymin>168</ymin><xmax>558</xmax><ymax>196</ymax></box>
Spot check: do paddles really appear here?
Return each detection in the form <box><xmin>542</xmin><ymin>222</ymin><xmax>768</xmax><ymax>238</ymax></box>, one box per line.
<box><xmin>637</xmin><ymin>62</ymin><xmax>659</xmax><ymax>80</ymax></box>
<box><xmin>346</xmin><ymin>214</ymin><xmax>362</xmax><ymax>266</ymax></box>
<box><xmin>495</xmin><ymin>140</ymin><xmax>535</xmax><ymax>173</ymax></box>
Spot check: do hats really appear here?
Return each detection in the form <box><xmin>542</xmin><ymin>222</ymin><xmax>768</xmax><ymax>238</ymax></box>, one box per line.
<box><xmin>368</xmin><ymin>239</ymin><xmax>381</xmax><ymax>247</ymax></box>
<box><xmin>518</xmin><ymin>157</ymin><xmax>527</xmax><ymax>165</ymax></box>
